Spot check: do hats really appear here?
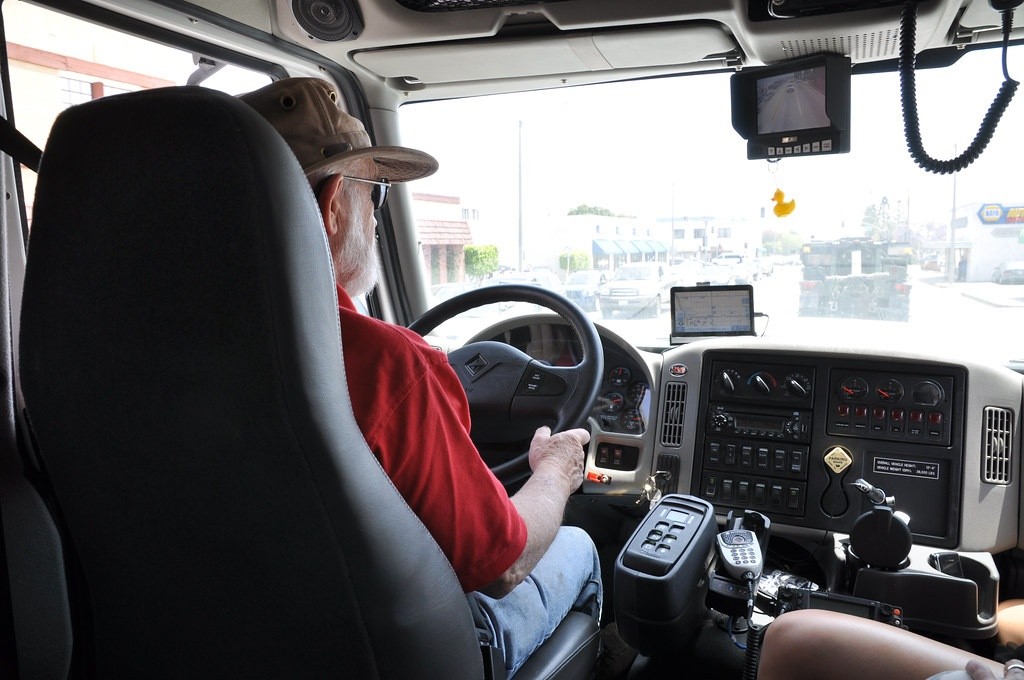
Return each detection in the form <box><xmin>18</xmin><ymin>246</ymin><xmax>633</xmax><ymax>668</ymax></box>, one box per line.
<box><xmin>238</xmin><ymin>77</ymin><xmax>439</xmax><ymax>184</ymax></box>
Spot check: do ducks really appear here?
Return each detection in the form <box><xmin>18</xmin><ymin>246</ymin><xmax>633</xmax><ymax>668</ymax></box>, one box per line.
<box><xmin>770</xmin><ymin>188</ymin><xmax>796</xmax><ymax>218</ymax></box>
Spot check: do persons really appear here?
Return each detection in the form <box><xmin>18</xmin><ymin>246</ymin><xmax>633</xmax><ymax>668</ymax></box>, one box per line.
<box><xmin>239</xmin><ymin>76</ymin><xmax>605</xmax><ymax>680</ymax></box>
<box><xmin>756</xmin><ymin>598</ymin><xmax>1024</xmax><ymax>679</ymax></box>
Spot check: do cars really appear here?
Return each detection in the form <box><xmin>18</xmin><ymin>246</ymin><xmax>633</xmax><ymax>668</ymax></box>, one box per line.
<box><xmin>421</xmin><ymin>264</ymin><xmax>601</xmax><ymax>324</ymax></box>
<box><xmin>673</xmin><ymin>222</ymin><xmax>1024</xmax><ymax>325</ymax></box>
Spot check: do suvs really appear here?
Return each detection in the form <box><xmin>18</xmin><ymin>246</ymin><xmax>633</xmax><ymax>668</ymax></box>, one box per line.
<box><xmin>597</xmin><ymin>260</ymin><xmax>676</xmax><ymax>318</ymax></box>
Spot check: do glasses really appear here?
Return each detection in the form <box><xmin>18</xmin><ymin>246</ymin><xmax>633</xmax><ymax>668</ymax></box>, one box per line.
<box><xmin>343</xmin><ymin>176</ymin><xmax>392</xmax><ymax>210</ymax></box>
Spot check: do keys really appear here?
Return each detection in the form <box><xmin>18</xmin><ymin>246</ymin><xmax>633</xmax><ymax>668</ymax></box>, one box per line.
<box><xmin>635</xmin><ymin>470</ymin><xmax>669</xmax><ymax>509</ymax></box>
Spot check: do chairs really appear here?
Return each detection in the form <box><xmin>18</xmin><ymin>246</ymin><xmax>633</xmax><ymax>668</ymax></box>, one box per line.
<box><xmin>21</xmin><ymin>85</ymin><xmax>604</xmax><ymax>680</ymax></box>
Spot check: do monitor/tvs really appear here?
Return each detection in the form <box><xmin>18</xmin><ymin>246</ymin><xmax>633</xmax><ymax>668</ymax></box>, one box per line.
<box><xmin>669</xmin><ymin>284</ymin><xmax>756</xmax><ymax>338</ymax></box>
<box><xmin>755</xmin><ymin>64</ymin><xmax>831</xmax><ymax>134</ymax></box>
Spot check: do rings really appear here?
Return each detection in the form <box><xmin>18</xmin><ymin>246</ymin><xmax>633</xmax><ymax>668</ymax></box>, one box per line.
<box><xmin>1005</xmin><ymin>664</ymin><xmax>1024</xmax><ymax>677</ymax></box>
